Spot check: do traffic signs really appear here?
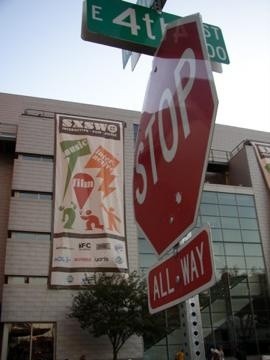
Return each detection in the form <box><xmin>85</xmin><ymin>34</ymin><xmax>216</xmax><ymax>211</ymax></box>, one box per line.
<box><xmin>145</xmin><ymin>223</ymin><xmax>216</xmax><ymax>314</ymax></box>
<box><xmin>81</xmin><ymin>0</ymin><xmax>229</xmax><ymax>65</ymax></box>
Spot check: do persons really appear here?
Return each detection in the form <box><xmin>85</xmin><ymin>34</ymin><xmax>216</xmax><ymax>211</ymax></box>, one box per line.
<box><xmin>210</xmin><ymin>347</ymin><xmax>224</xmax><ymax>360</ymax></box>
<box><xmin>80</xmin><ymin>355</ymin><xmax>85</xmax><ymax>360</ymax></box>
<box><xmin>235</xmin><ymin>346</ymin><xmax>246</xmax><ymax>360</ymax></box>
<box><xmin>176</xmin><ymin>350</ymin><xmax>185</xmax><ymax>360</ymax></box>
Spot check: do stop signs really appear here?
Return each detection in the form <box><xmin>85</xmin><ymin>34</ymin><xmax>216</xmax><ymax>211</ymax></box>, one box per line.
<box><xmin>131</xmin><ymin>13</ymin><xmax>219</xmax><ymax>260</ymax></box>
